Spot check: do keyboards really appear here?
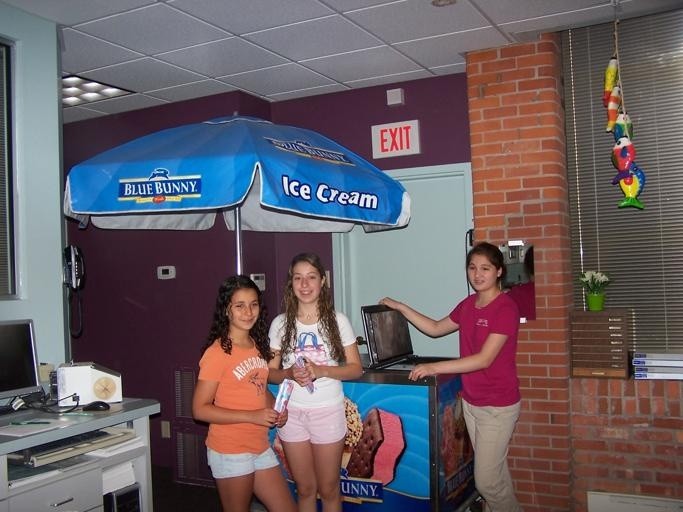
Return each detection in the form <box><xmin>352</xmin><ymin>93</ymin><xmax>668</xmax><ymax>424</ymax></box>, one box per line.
<box><xmin>15</xmin><ymin>429</ymin><xmax>125</xmax><ymax>460</ymax></box>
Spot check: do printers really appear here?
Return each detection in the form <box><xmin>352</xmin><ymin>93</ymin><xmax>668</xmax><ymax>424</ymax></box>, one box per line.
<box><xmin>57</xmin><ymin>361</ymin><xmax>123</xmax><ymax>407</ymax></box>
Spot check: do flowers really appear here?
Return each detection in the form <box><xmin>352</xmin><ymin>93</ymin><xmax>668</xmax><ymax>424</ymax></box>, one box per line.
<box><xmin>578</xmin><ymin>271</ymin><xmax>612</xmax><ymax>293</ymax></box>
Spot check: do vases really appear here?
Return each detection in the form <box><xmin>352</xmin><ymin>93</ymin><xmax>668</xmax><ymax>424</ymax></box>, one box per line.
<box><xmin>586</xmin><ymin>293</ymin><xmax>605</xmax><ymax>311</ymax></box>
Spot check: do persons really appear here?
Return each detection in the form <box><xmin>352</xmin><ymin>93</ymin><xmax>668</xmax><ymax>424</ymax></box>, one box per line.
<box><xmin>380</xmin><ymin>241</ymin><xmax>524</xmax><ymax>512</ymax></box>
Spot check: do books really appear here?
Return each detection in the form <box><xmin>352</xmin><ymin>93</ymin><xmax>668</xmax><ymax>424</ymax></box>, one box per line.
<box><xmin>0</xmin><ymin>417</ymin><xmax>69</xmax><ymax>438</ymax></box>
<box><xmin>88</xmin><ymin>439</ymin><xmax>146</xmax><ymax>457</ymax></box>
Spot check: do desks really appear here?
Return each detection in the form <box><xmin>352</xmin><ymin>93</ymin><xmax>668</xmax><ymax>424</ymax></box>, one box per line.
<box><xmin>0</xmin><ymin>392</ymin><xmax>163</xmax><ymax>511</ymax></box>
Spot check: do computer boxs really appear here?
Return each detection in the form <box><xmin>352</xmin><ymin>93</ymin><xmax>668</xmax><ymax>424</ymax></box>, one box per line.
<box><xmin>104</xmin><ymin>483</ymin><xmax>141</xmax><ymax>512</ymax></box>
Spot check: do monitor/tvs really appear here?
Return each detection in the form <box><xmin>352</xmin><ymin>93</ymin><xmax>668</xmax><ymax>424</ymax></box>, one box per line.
<box><xmin>0</xmin><ymin>320</ymin><xmax>43</xmax><ymax>415</ymax></box>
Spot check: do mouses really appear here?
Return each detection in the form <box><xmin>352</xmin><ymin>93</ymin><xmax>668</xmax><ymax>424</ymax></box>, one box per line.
<box><xmin>83</xmin><ymin>402</ymin><xmax>109</xmax><ymax>411</ymax></box>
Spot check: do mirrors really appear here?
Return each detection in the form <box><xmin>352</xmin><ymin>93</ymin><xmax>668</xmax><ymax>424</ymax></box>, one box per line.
<box><xmin>499</xmin><ymin>242</ymin><xmax>536</xmax><ymax>323</ymax></box>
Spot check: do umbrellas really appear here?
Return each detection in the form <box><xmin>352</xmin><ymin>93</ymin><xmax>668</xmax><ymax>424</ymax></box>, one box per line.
<box><xmin>61</xmin><ymin>112</ymin><xmax>411</xmax><ymax>273</ymax></box>
